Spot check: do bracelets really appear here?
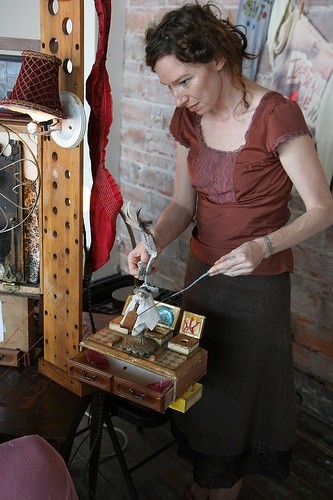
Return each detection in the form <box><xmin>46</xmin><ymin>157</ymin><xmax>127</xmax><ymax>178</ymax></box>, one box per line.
<box><xmin>260</xmin><ymin>234</ymin><xmax>277</xmax><ymax>256</ymax></box>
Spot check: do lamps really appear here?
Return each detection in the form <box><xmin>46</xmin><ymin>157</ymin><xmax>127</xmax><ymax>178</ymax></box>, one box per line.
<box><xmin>1</xmin><ymin>50</ymin><xmax>86</xmax><ymax>150</ymax></box>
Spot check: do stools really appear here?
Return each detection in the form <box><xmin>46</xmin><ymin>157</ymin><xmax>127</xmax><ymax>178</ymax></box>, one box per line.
<box><xmin>60</xmin><ymin>391</ymin><xmax>194</xmax><ymax>500</ymax></box>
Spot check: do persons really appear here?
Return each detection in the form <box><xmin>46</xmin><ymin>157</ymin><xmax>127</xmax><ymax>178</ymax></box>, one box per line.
<box><xmin>126</xmin><ymin>0</ymin><xmax>333</xmax><ymax>500</ymax></box>
